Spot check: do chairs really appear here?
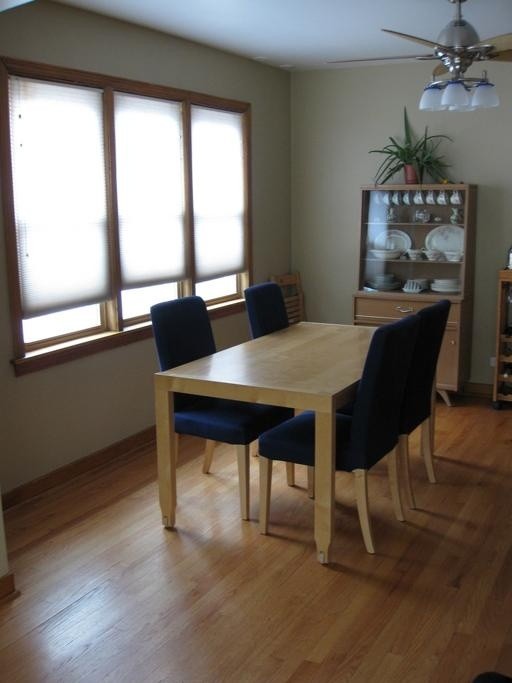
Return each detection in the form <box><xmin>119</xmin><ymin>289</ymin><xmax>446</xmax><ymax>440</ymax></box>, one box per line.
<box><xmin>401</xmin><ymin>299</ymin><xmax>451</xmax><ymax>509</ymax></box>
<box><xmin>270</xmin><ymin>270</ymin><xmax>305</xmax><ymax>325</ymax></box>
<box><xmin>256</xmin><ymin>311</ymin><xmax>424</xmax><ymax>553</ymax></box>
<box><xmin>150</xmin><ymin>296</ymin><xmax>294</xmax><ymax>520</ymax></box>
<box><xmin>243</xmin><ymin>283</ymin><xmax>288</xmax><ymax>338</ymax></box>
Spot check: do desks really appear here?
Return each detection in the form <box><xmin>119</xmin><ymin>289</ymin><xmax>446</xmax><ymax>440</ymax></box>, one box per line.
<box><xmin>154</xmin><ymin>321</ymin><xmax>379</xmax><ymax>564</ymax></box>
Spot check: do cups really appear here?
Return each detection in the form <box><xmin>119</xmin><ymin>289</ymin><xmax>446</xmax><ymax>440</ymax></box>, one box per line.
<box><xmin>371</xmin><ymin>189</ymin><xmax>463</xmax><ymax>205</ymax></box>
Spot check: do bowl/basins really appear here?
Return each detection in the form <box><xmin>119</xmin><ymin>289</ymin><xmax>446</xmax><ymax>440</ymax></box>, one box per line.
<box><xmin>369</xmin><ymin>249</ymin><xmax>401</xmax><ymax>259</ymax></box>
<box><xmin>406</xmin><ymin>249</ymin><xmax>461</xmax><ymax>262</ymax></box>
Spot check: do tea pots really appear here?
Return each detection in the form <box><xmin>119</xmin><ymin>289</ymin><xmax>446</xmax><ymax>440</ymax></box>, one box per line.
<box><xmin>449</xmin><ymin>208</ymin><xmax>465</xmax><ymax>225</ymax></box>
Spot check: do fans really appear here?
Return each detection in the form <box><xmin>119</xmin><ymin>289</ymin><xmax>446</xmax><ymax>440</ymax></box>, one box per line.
<box><xmin>326</xmin><ymin>0</ymin><xmax>512</xmax><ymax>78</ymax></box>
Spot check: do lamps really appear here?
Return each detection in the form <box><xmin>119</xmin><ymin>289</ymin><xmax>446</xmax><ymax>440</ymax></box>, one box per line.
<box><xmin>420</xmin><ymin>79</ymin><xmax>499</xmax><ymax>112</ymax></box>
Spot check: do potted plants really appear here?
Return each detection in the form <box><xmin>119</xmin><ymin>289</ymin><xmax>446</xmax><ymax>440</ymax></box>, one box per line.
<box><xmin>369</xmin><ymin>105</ymin><xmax>451</xmax><ymax>185</ymax></box>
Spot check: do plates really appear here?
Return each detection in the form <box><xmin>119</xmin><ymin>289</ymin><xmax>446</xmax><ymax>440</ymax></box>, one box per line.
<box><xmin>367</xmin><ymin>273</ymin><xmax>460</xmax><ymax>293</ymax></box>
<box><xmin>374</xmin><ymin>229</ymin><xmax>412</xmax><ymax>255</ymax></box>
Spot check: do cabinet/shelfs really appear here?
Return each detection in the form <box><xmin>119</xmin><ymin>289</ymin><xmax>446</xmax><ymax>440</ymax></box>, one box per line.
<box><xmin>355</xmin><ymin>186</ymin><xmax>474</xmax><ymax>406</ymax></box>
<box><xmin>493</xmin><ymin>270</ymin><xmax>512</xmax><ymax>402</ymax></box>
